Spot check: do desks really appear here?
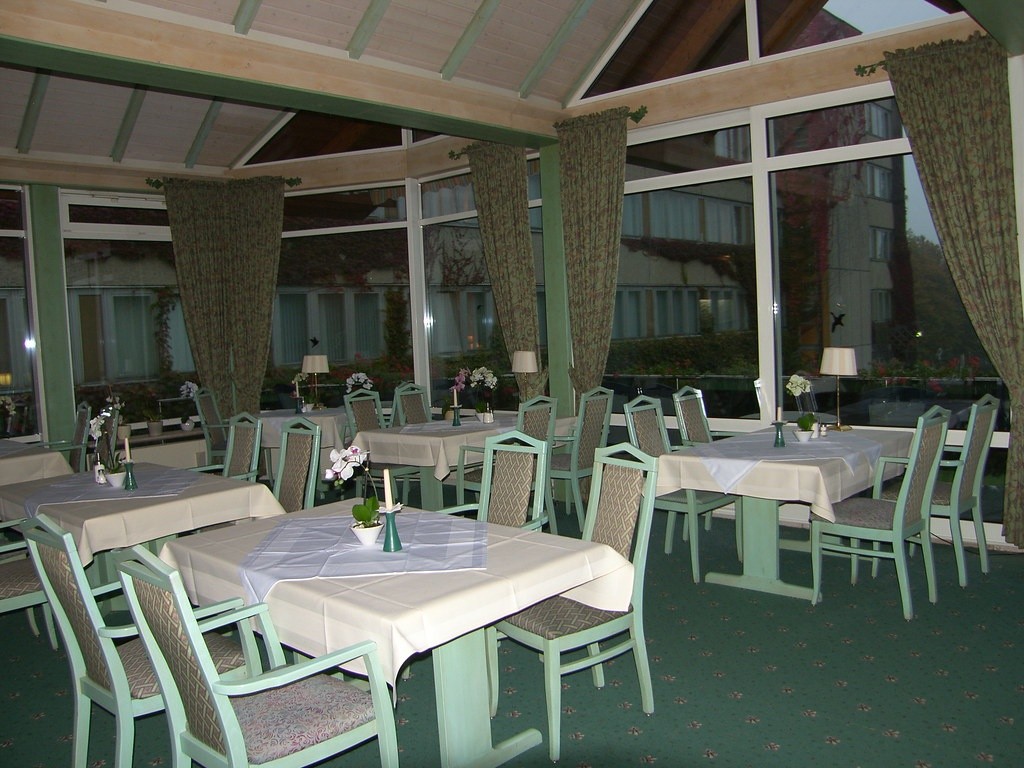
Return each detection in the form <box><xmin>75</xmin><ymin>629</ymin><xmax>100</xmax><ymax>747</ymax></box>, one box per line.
<box><xmin>654</xmin><ymin>424</ymin><xmax>913</xmax><ymax>605</ymax></box>
<box><xmin>350</xmin><ymin>413</ymin><xmax>523</xmax><ymax>512</ymax></box>
<box><xmin>255</xmin><ymin>407</ymin><xmax>346</xmax><ymax>478</ymax></box>
<box><xmin>160</xmin><ymin>497</ymin><xmax>636</xmax><ymax>768</ymax></box>
<box><xmin>0</xmin><ymin>463</ymin><xmax>284</xmax><ymax>630</ymax></box>
<box><xmin>0</xmin><ymin>439</ymin><xmax>66</xmax><ymax>491</ymax></box>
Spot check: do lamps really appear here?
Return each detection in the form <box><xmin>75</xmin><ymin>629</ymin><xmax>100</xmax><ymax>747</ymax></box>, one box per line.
<box><xmin>301</xmin><ymin>353</ymin><xmax>329</xmax><ymax>411</ymax></box>
<box><xmin>820</xmin><ymin>347</ymin><xmax>859</xmax><ymax>430</ymax></box>
<box><xmin>512</xmin><ymin>349</ymin><xmax>536</xmax><ymax>402</ymax></box>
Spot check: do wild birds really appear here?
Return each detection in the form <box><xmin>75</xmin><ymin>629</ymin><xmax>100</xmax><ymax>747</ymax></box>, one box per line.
<box><xmin>829</xmin><ymin>312</ymin><xmax>845</xmax><ymax>333</ymax></box>
<box><xmin>309</xmin><ymin>337</ymin><xmax>319</xmax><ymax>348</ymax></box>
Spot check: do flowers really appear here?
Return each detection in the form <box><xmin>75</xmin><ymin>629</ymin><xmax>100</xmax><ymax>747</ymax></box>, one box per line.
<box><xmin>90</xmin><ymin>410</ymin><xmax>126</xmax><ymax>472</ymax></box>
<box><xmin>345</xmin><ymin>371</ymin><xmax>373</xmax><ymax>392</ymax></box>
<box><xmin>105</xmin><ymin>383</ymin><xmax>131</xmax><ymax>426</ymax></box>
<box><xmin>450</xmin><ymin>367</ymin><xmax>470</xmax><ymax>391</ymax></box>
<box><xmin>293</xmin><ymin>371</ymin><xmax>317</xmax><ymax>403</ymax></box>
<box><xmin>0</xmin><ymin>395</ymin><xmax>14</xmax><ymax>418</ymax></box>
<box><xmin>327</xmin><ymin>444</ymin><xmax>382</xmax><ymax>521</ymax></box>
<box><xmin>788</xmin><ymin>376</ymin><xmax>813</xmax><ymax>400</ymax></box>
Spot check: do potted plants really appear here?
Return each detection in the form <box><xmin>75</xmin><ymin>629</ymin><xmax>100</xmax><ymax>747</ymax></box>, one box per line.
<box><xmin>141</xmin><ymin>408</ymin><xmax>164</xmax><ymax>437</ymax></box>
<box><xmin>795</xmin><ymin>414</ymin><xmax>814</xmax><ymax>441</ymax></box>
<box><xmin>179</xmin><ymin>414</ymin><xmax>195</xmax><ymax>431</ymax></box>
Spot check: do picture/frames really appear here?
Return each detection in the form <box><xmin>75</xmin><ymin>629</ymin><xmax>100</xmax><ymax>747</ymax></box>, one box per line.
<box><xmin>180</xmin><ymin>381</ymin><xmax>200</xmax><ymax>400</ymax></box>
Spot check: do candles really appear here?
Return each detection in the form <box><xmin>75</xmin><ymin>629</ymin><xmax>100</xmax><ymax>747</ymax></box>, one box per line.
<box><xmin>777</xmin><ymin>405</ymin><xmax>783</xmax><ymax>422</ymax></box>
<box><xmin>125</xmin><ymin>437</ymin><xmax>133</xmax><ymax>463</ymax></box>
<box><xmin>382</xmin><ymin>467</ymin><xmax>395</xmax><ymax>510</ymax></box>
<box><xmin>453</xmin><ymin>389</ymin><xmax>457</xmax><ymax>406</ymax></box>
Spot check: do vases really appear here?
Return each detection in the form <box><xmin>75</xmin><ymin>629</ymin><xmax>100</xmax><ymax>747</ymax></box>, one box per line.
<box><xmin>117</xmin><ymin>426</ymin><xmax>131</xmax><ymax>441</ymax></box>
<box><xmin>348</xmin><ymin>519</ymin><xmax>384</xmax><ymax>546</ymax></box>
<box><xmin>105</xmin><ymin>471</ymin><xmax>127</xmax><ymax>488</ymax></box>
<box><xmin>471</xmin><ymin>368</ymin><xmax>497</xmax><ymax>390</ymax></box>
<box><xmin>306</xmin><ymin>402</ymin><xmax>314</xmax><ymax>410</ymax></box>
<box><xmin>472</xmin><ymin>409</ymin><xmax>495</xmax><ymax>424</ymax></box>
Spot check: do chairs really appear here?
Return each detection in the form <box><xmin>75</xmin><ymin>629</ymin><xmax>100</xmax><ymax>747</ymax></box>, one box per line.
<box><xmin>0</xmin><ymin>379</ymin><xmax>1003</xmax><ymax>768</ymax></box>
<box><xmin>437</xmin><ymin>433</ymin><xmax>551</xmax><ymax>534</ymax></box>
<box><xmin>110</xmin><ymin>546</ymin><xmax>401</xmax><ymax>767</ymax></box>
<box><xmin>96</xmin><ymin>406</ymin><xmax>121</xmax><ymax>466</ymax></box>
